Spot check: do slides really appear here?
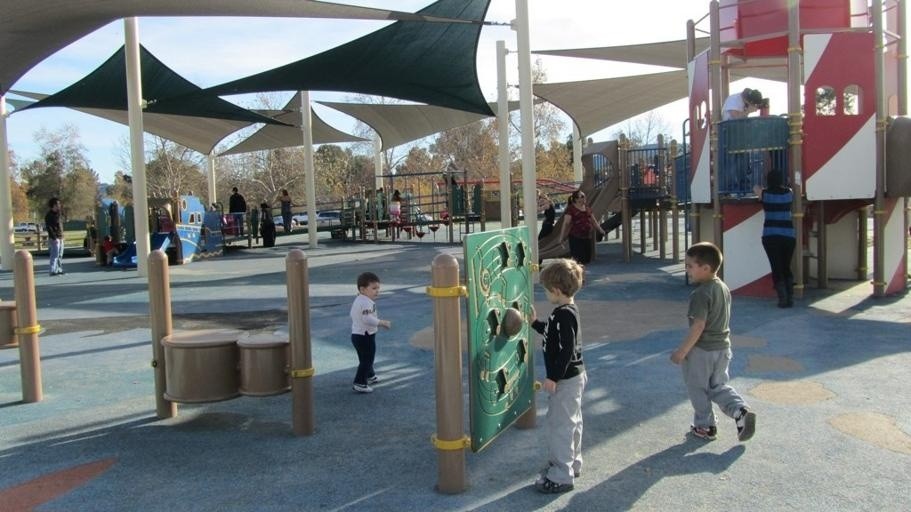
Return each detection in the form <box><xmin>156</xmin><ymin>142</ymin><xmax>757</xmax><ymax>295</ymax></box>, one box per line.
<box><xmin>538</xmin><ymin>140</ymin><xmax>619</xmax><ymax>259</ymax></box>
<box><xmin>112</xmin><ymin>232</ymin><xmax>171</xmax><ymax>269</ymax></box>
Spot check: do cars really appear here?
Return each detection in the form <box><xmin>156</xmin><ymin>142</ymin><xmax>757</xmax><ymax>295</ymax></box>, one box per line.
<box><xmin>14</xmin><ymin>222</ymin><xmax>43</xmax><ymax>233</ymax></box>
<box><xmin>271</xmin><ymin>208</ymin><xmax>346</xmax><ymax>227</ymax></box>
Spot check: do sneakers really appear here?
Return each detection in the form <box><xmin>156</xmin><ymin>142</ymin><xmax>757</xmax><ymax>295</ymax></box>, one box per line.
<box><xmin>368</xmin><ymin>375</ymin><xmax>381</xmax><ymax>384</ymax></box>
<box><xmin>536</xmin><ymin>476</ymin><xmax>574</xmax><ymax>492</ymax></box>
<box><xmin>734</xmin><ymin>408</ymin><xmax>755</xmax><ymax>441</ymax></box>
<box><xmin>779</xmin><ymin>301</ymin><xmax>792</xmax><ymax>308</ymax></box>
<box><xmin>353</xmin><ymin>383</ymin><xmax>374</xmax><ymax>392</ymax></box>
<box><xmin>691</xmin><ymin>424</ymin><xmax>717</xmax><ymax>440</ymax></box>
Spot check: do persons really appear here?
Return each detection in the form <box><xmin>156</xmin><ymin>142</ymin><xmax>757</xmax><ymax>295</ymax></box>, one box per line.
<box><xmin>761</xmin><ymin>168</ymin><xmax>796</xmax><ymax>309</ymax></box>
<box><xmin>346</xmin><ymin>272</ymin><xmax>391</xmax><ymax>394</ymax></box>
<box><xmin>101</xmin><ymin>235</ymin><xmax>119</xmax><ymax>265</ymax></box>
<box><xmin>272</xmin><ymin>188</ymin><xmax>299</xmax><ymax>236</ymax></box>
<box><xmin>560</xmin><ymin>189</ymin><xmax>607</xmax><ymax>273</ymax></box>
<box><xmin>672</xmin><ymin>237</ymin><xmax>757</xmax><ymax>445</ymax></box>
<box><xmin>228</xmin><ymin>187</ymin><xmax>247</xmax><ymax>238</ymax></box>
<box><xmin>44</xmin><ymin>197</ymin><xmax>65</xmax><ymax>276</ymax></box>
<box><xmin>722</xmin><ymin>88</ymin><xmax>765</xmax><ymax>198</ymax></box>
<box><xmin>528</xmin><ymin>258</ymin><xmax>586</xmax><ymax>495</ymax></box>
